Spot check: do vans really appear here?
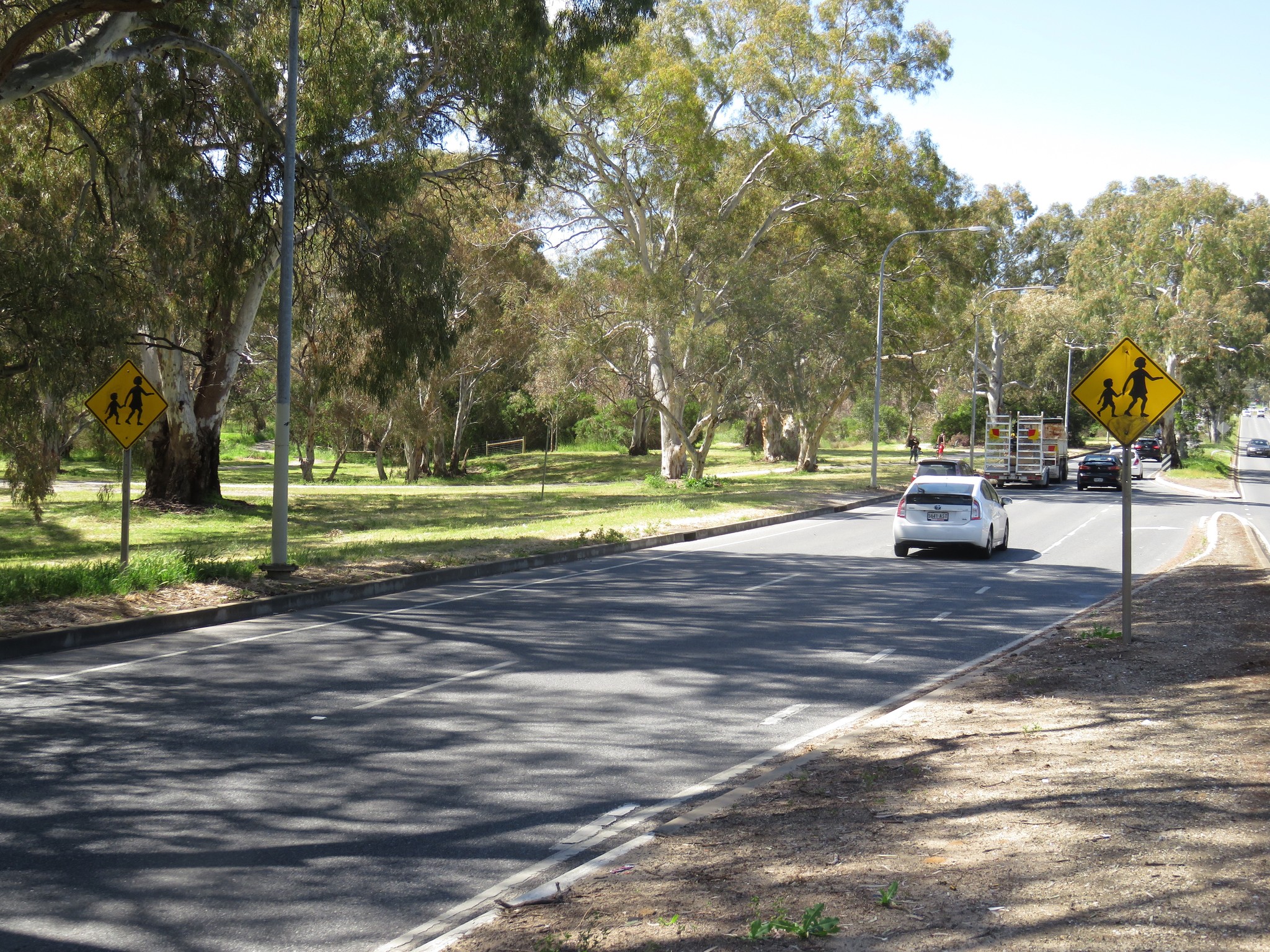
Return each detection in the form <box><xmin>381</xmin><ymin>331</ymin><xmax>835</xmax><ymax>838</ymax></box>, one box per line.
<box><xmin>1257</xmin><ymin>410</ymin><xmax>1265</xmax><ymax>418</ymax></box>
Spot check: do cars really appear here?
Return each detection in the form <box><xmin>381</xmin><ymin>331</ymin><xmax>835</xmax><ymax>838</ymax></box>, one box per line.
<box><xmin>1077</xmin><ymin>451</ymin><xmax>1122</xmax><ymax>491</ymax></box>
<box><xmin>1243</xmin><ymin>411</ymin><xmax>1252</xmax><ymax>417</ymax></box>
<box><xmin>1247</xmin><ymin>405</ymin><xmax>1256</xmax><ymax>409</ymax></box>
<box><xmin>1108</xmin><ymin>448</ymin><xmax>1146</xmax><ymax>479</ymax></box>
<box><xmin>911</xmin><ymin>458</ymin><xmax>978</xmax><ymax>494</ymax></box>
<box><xmin>1154</xmin><ymin>430</ymin><xmax>1162</xmax><ymax>440</ymax></box>
<box><xmin>1245</xmin><ymin>438</ymin><xmax>1270</xmax><ymax>457</ymax></box>
<box><xmin>1134</xmin><ymin>437</ymin><xmax>1164</xmax><ymax>462</ymax></box>
<box><xmin>892</xmin><ymin>476</ymin><xmax>1013</xmax><ymax>558</ymax></box>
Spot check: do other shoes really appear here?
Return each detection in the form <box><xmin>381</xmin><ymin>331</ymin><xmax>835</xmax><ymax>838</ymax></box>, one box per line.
<box><xmin>909</xmin><ymin>462</ymin><xmax>911</xmax><ymax>464</ymax></box>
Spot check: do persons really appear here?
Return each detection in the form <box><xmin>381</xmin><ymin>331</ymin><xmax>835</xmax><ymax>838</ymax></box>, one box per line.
<box><xmin>936</xmin><ymin>442</ymin><xmax>945</xmax><ymax>459</ymax></box>
<box><xmin>937</xmin><ymin>430</ymin><xmax>946</xmax><ymax>459</ymax></box>
<box><xmin>908</xmin><ymin>435</ymin><xmax>919</xmax><ymax>465</ymax></box>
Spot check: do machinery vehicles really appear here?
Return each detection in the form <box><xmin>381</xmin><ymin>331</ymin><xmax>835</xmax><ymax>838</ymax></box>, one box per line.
<box><xmin>982</xmin><ymin>410</ymin><xmax>1073</xmax><ymax>488</ymax></box>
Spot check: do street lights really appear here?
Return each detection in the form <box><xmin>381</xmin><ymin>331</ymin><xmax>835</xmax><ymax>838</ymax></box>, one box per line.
<box><xmin>869</xmin><ymin>227</ymin><xmax>990</xmax><ymax>488</ymax></box>
<box><xmin>968</xmin><ymin>286</ymin><xmax>1058</xmax><ymax>468</ymax></box>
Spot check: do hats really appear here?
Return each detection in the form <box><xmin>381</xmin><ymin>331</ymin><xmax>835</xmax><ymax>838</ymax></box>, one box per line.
<box><xmin>942</xmin><ymin>430</ymin><xmax>945</xmax><ymax>433</ymax></box>
<box><xmin>911</xmin><ymin>436</ymin><xmax>916</xmax><ymax>438</ymax></box>
